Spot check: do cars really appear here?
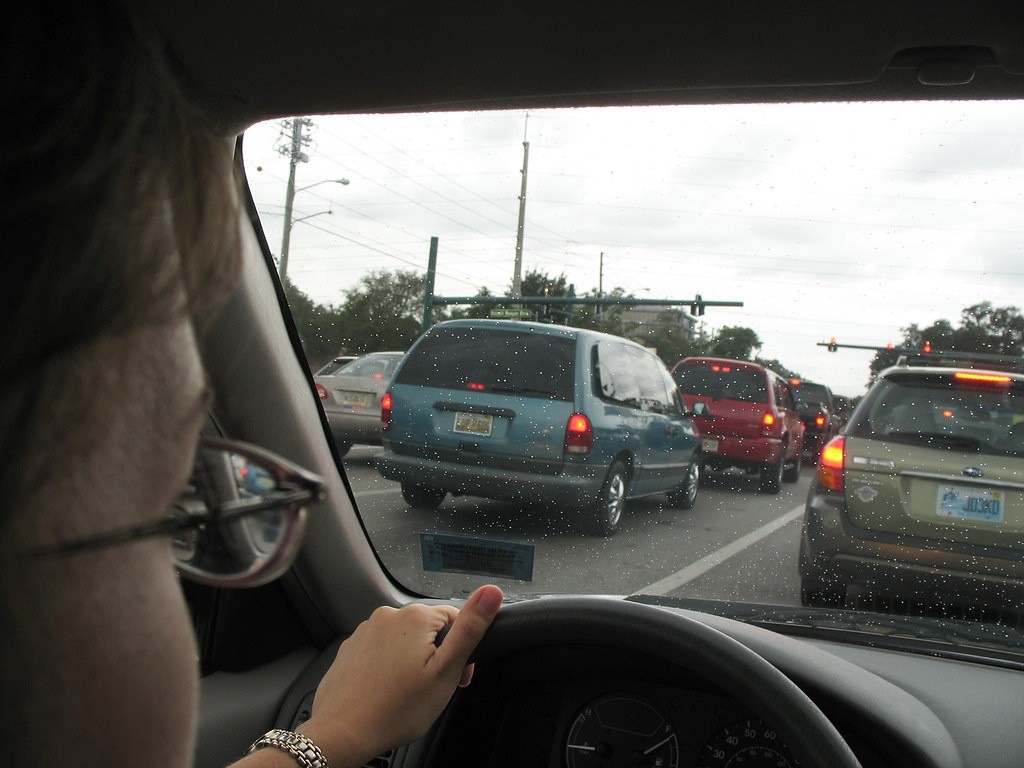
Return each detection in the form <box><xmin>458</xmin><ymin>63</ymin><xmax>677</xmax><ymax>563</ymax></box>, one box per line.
<box><xmin>310</xmin><ymin>351</ymin><xmax>408</xmax><ymax>458</ymax></box>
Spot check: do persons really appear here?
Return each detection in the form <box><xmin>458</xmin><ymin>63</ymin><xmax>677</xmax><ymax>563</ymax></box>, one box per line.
<box><xmin>1</xmin><ymin>0</ymin><xmax>502</xmax><ymax>768</ymax></box>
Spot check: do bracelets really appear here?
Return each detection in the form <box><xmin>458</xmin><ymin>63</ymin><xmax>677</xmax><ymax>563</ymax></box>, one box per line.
<box><xmin>245</xmin><ymin>728</ymin><xmax>329</xmax><ymax>768</ymax></box>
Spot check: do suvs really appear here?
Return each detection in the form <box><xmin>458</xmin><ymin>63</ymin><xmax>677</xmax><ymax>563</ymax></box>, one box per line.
<box><xmin>785</xmin><ymin>380</ymin><xmax>856</xmax><ymax>465</ymax></box>
<box><xmin>372</xmin><ymin>314</ymin><xmax>712</xmax><ymax>538</ymax></box>
<box><xmin>798</xmin><ymin>355</ymin><xmax>1023</xmax><ymax>627</ymax></box>
<box><xmin>668</xmin><ymin>355</ymin><xmax>807</xmax><ymax>495</ymax></box>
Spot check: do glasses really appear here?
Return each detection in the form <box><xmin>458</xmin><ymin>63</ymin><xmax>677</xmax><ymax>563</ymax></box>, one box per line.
<box><xmin>0</xmin><ymin>434</ymin><xmax>327</xmax><ymax>589</ymax></box>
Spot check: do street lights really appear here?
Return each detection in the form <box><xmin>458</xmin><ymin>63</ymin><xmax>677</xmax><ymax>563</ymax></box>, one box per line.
<box><xmin>279</xmin><ymin>177</ymin><xmax>350</xmax><ymax>280</ymax></box>
